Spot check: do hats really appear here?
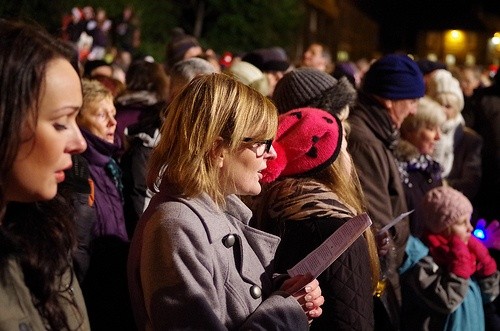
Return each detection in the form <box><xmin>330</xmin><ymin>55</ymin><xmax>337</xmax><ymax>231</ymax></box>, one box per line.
<box><xmin>167</xmin><ymin>26</ymin><xmax>201</xmax><ymax>65</ymax></box>
<box><xmin>257</xmin><ymin>107</ymin><xmax>343</xmax><ymax>184</ymax></box>
<box><xmin>362</xmin><ymin>54</ymin><xmax>425</xmax><ymax>99</ymax></box>
<box><xmin>427</xmin><ymin>69</ymin><xmax>464</xmax><ymax>110</ymax></box>
<box><xmin>420</xmin><ymin>186</ymin><xmax>473</xmax><ymax>233</ymax></box>
<box><xmin>272</xmin><ymin>68</ymin><xmax>357</xmax><ymax>115</ymax></box>
<box><xmin>241</xmin><ymin>46</ymin><xmax>289</xmax><ymax>73</ymax></box>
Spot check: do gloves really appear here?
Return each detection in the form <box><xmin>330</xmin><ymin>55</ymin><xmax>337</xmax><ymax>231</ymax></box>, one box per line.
<box><xmin>441</xmin><ymin>234</ymin><xmax>479</xmax><ymax>279</ymax></box>
<box><xmin>465</xmin><ymin>235</ymin><xmax>496</xmax><ymax>277</ymax></box>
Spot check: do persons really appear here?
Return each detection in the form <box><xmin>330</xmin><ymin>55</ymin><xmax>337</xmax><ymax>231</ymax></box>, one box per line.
<box><xmin>0</xmin><ymin>6</ymin><xmax>500</xmax><ymax>331</ymax></box>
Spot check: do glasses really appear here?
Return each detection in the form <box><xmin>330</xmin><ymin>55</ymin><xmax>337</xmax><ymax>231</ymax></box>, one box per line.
<box><xmin>242</xmin><ymin>137</ymin><xmax>274</xmax><ymax>157</ymax></box>
